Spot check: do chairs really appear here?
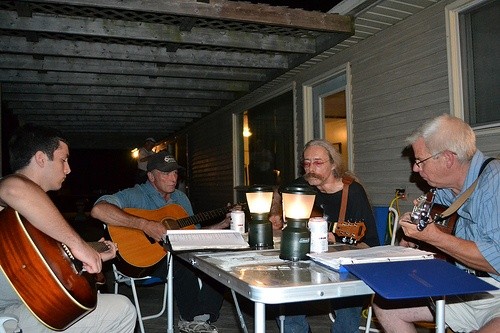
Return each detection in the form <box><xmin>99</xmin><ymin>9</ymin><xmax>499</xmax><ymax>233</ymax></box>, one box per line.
<box><xmin>104</xmin><ymin>224</ymin><xmax>168</xmax><ymax>333</ymax></box>
<box><xmin>329</xmin><ymin>206</ymin><xmax>398</xmax><ymax>333</ymax></box>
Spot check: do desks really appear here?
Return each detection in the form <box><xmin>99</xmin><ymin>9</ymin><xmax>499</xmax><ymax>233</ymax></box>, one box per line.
<box><xmin>165</xmin><ymin>235</ymin><xmax>446</xmax><ymax>333</ymax></box>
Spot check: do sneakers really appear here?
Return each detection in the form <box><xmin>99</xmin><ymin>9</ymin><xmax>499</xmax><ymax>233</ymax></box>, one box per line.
<box><xmin>178</xmin><ymin>314</ymin><xmax>218</xmax><ymax>333</ymax></box>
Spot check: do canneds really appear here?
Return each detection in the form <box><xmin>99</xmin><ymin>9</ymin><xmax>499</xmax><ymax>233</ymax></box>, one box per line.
<box><xmin>230</xmin><ymin>211</ymin><xmax>245</xmax><ymax>233</ymax></box>
<box><xmin>308</xmin><ymin>217</ymin><xmax>329</xmax><ymax>252</ymax></box>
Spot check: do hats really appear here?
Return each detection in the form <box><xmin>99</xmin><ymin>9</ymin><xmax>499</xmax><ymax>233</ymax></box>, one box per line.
<box><xmin>147</xmin><ymin>151</ymin><xmax>187</xmax><ymax>173</ymax></box>
<box><xmin>411</xmin><ymin>150</ymin><xmax>457</xmax><ymax>168</ymax></box>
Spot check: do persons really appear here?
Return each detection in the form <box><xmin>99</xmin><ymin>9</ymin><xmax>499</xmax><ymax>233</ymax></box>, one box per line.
<box><xmin>276</xmin><ymin>138</ymin><xmax>380</xmax><ymax>333</ymax></box>
<box><xmin>371</xmin><ymin>113</ymin><xmax>500</xmax><ymax>333</ymax></box>
<box><xmin>90</xmin><ymin>151</ymin><xmax>244</xmax><ymax>333</ymax></box>
<box><xmin>0</xmin><ymin>122</ymin><xmax>138</xmax><ymax>333</ymax></box>
<box><xmin>138</xmin><ymin>137</ymin><xmax>158</xmax><ymax>185</ymax></box>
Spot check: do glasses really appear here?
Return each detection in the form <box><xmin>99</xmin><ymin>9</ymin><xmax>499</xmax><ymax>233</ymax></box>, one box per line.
<box><xmin>302</xmin><ymin>158</ymin><xmax>332</xmax><ymax>168</ymax></box>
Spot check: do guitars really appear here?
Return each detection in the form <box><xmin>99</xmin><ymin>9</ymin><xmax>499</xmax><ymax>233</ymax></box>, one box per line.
<box><xmin>409</xmin><ymin>191</ymin><xmax>456</xmax><ymax>261</ymax></box>
<box><xmin>0</xmin><ymin>206</ymin><xmax>111</xmax><ymax>332</ymax></box>
<box><xmin>103</xmin><ymin>201</ymin><xmax>249</xmax><ymax>278</ymax></box>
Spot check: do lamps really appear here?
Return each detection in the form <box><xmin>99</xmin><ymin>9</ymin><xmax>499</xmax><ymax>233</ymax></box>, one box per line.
<box><xmin>244</xmin><ymin>177</ymin><xmax>318</xmax><ymax>262</ymax></box>
